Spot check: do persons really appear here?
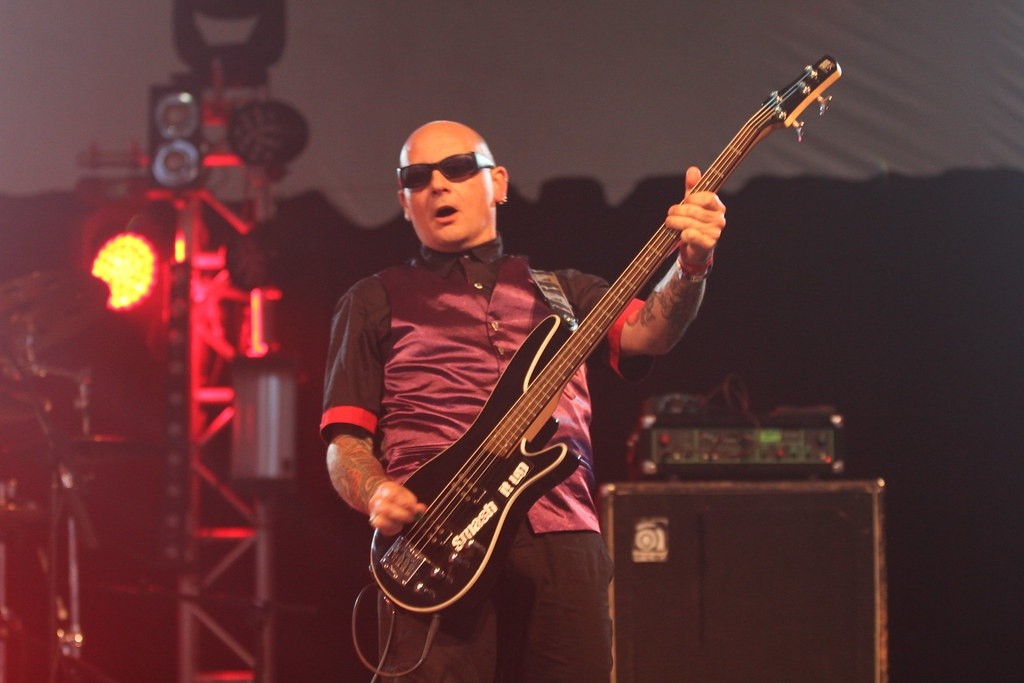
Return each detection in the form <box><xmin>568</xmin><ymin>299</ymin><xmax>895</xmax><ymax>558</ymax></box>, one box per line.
<box><xmin>318</xmin><ymin>121</ymin><xmax>727</xmax><ymax>682</ymax></box>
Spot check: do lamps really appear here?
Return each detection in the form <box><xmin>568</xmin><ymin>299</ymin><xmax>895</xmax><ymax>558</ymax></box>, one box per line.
<box><xmin>146</xmin><ymin>85</ymin><xmax>207</xmax><ymax>191</ymax></box>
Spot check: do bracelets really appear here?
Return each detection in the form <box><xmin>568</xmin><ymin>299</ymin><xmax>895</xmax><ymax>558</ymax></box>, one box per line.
<box><xmin>676</xmin><ymin>255</ymin><xmax>714</xmax><ymax>282</ymax></box>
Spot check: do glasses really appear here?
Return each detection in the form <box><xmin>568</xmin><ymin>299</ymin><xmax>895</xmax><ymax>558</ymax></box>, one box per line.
<box><xmin>397</xmin><ymin>152</ymin><xmax>495</xmax><ymax>191</ymax></box>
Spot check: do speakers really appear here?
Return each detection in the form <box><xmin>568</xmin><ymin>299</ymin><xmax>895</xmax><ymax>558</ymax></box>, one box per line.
<box><xmin>594</xmin><ymin>478</ymin><xmax>888</xmax><ymax>683</ymax></box>
<box><xmin>146</xmin><ymin>84</ymin><xmax>206</xmax><ymax>190</ymax></box>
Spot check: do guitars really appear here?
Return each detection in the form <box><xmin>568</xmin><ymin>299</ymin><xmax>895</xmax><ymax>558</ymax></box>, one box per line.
<box><xmin>368</xmin><ymin>52</ymin><xmax>845</xmax><ymax>618</ymax></box>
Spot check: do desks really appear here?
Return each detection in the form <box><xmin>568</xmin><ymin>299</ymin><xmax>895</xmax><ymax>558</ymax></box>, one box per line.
<box><xmin>591</xmin><ymin>478</ymin><xmax>887</xmax><ymax>683</ymax></box>
